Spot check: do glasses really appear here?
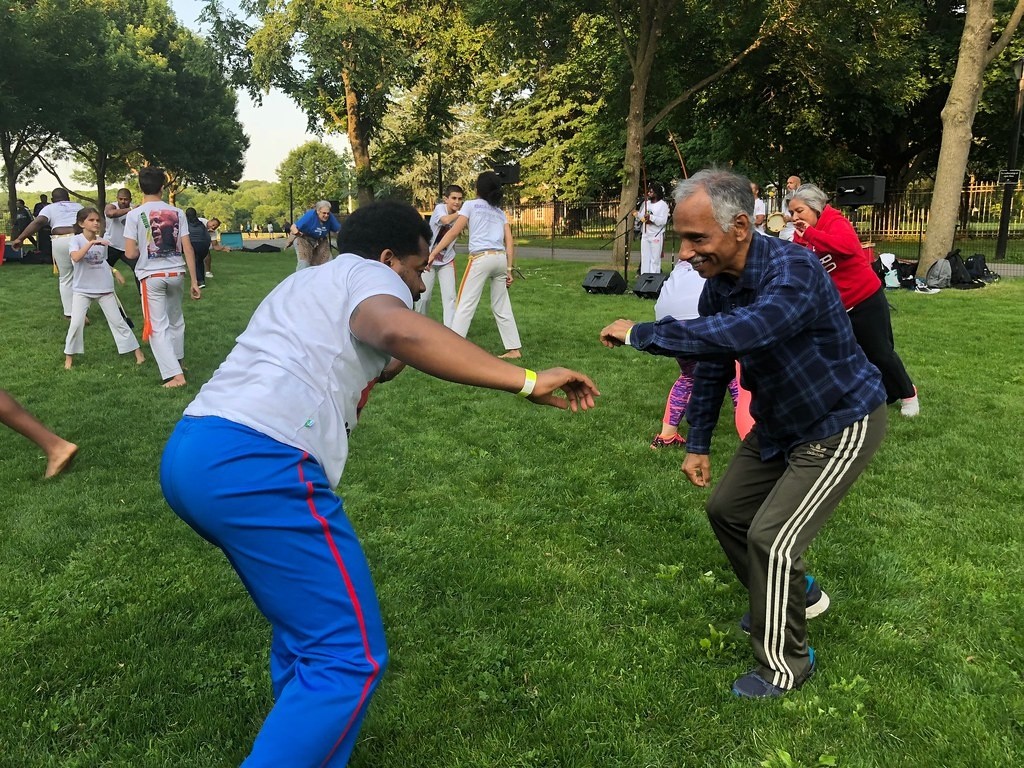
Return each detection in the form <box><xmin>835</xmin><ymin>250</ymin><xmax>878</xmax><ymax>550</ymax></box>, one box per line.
<box><xmin>320</xmin><ymin>211</ymin><xmax>330</xmax><ymax>215</ymax></box>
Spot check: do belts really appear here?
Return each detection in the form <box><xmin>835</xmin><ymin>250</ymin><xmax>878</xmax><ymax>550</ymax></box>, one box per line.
<box><xmin>152</xmin><ymin>272</ymin><xmax>185</xmax><ymax>277</ymax></box>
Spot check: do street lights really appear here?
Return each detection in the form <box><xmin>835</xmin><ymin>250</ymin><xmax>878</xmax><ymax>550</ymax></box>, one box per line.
<box><xmin>288</xmin><ymin>175</ymin><xmax>294</xmax><ymax>226</ymax></box>
<box><xmin>991</xmin><ymin>55</ymin><xmax>1024</xmax><ymax>259</ymax></box>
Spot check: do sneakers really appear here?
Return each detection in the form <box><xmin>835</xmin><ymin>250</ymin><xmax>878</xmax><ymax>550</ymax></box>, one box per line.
<box><xmin>741</xmin><ymin>575</ymin><xmax>830</xmax><ymax>636</ymax></box>
<box><xmin>900</xmin><ymin>384</ymin><xmax>919</xmax><ymax>417</ymax></box>
<box><xmin>649</xmin><ymin>433</ymin><xmax>685</xmax><ymax>450</ymax></box>
<box><xmin>915</xmin><ymin>283</ymin><xmax>940</xmax><ymax>295</ymax></box>
<box><xmin>733</xmin><ymin>647</ymin><xmax>817</xmax><ymax>698</ymax></box>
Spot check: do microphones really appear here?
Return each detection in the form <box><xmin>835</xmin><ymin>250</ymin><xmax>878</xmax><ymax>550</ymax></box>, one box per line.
<box><xmin>636</xmin><ymin>197</ymin><xmax>652</xmax><ymax>200</ymax></box>
<box><xmin>760</xmin><ymin>192</ymin><xmax>774</xmax><ymax>200</ymax></box>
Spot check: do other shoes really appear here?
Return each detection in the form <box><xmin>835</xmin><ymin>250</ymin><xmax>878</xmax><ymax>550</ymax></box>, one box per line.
<box><xmin>205</xmin><ymin>272</ymin><xmax>214</xmax><ymax>277</ymax></box>
<box><xmin>198</xmin><ymin>281</ymin><xmax>205</xmax><ymax>289</ymax></box>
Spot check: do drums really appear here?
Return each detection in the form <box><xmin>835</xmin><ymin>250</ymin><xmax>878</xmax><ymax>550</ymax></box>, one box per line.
<box><xmin>767</xmin><ymin>212</ymin><xmax>787</xmax><ymax>232</ymax></box>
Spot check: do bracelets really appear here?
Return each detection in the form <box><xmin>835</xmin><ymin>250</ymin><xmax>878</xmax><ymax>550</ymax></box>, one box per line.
<box><xmin>514</xmin><ymin>368</ymin><xmax>537</xmax><ymax>398</ymax></box>
<box><xmin>113</xmin><ymin>269</ymin><xmax>118</xmax><ymax>277</ymax></box>
<box><xmin>507</xmin><ymin>267</ymin><xmax>512</xmax><ymax>271</ymax></box>
<box><xmin>625</xmin><ymin>326</ymin><xmax>633</xmax><ymax>346</ymax></box>
<box><xmin>129</xmin><ymin>206</ymin><xmax>133</xmax><ymax>211</ymax></box>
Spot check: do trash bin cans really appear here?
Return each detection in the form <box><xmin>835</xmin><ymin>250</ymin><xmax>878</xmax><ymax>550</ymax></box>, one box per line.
<box><xmin>221</xmin><ymin>229</ymin><xmax>243</xmax><ymax>251</ymax></box>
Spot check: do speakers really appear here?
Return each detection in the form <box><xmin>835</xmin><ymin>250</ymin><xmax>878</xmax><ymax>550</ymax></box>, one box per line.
<box><xmin>582</xmin><ymin>269</ymin><xmax>627</xmax><ymax>296</ymax></box>
<box><xmin>493</xmin><ymin>164</ymin><xmax>520</xmax><ymax>184</ymax></box>
<box><xmin>633</xmin><ymin>273</ymin><xmax>670</xmax><ymax>300</ymax></box>
<box><xmin>835</xmin><ymin>174</ymin><xmax>886</xmax><ymax>204</ymax></box>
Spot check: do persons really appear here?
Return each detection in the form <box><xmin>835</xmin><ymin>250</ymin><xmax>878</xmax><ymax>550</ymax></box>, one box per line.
<box><xmin>290</xmin><ymin>201</ymin><xmax>341</xmax><ymax>272</ymax></box>
<box><xmin>281</xmin><ymin>218</ymin><xmax>291</xmax><ymax>238</ymax></box>
<box><xmin>750</xmin><ymin>183</ymin><xmax>765</xmax><ymax>236</ymax></box>
<box><xmin>779</xmin><ymin>176</ymin><xmax>801</xmax><ymax>242</ymax></box>
<box><xmin>425</xmin><ymin>171</ymin><xmax>523</xmax><ymax>359</ymax></box>
<box><xmin>160</xmin><ymin>199</ymin><xmax>601</xmax><ymax>768</ymax></box>
<box><xmin>267</xmin><ymin>221</ymin><xmax>275</xmax><ymax>240</ymax></box>
<box><xmin>638</xmin><ymin>182</ymin><xmax>669</xmax><ymax>276</ymax></box>
<box><xmin>415</xmin><ymin>185</ymin><xmax>463</xmax><ymax>330</ymax></box>
<box><xmin>785</xmin><ymin>183</ymin><xmax>919</xmax><ymax>418</ymax></box>
<box><xmin>0</xmin><ymin>387</ymin><xmax>79</xmax><ymax>480</ymax></box>
<box><xmin>649</xmin><ymin>259</ymin><xmax>755</xmax><ymax>450</ymax></box>
<box><xmin>11</xmin><ymin>165</ymin><xmax>230</xmax><ymax>388</ymax></box>
<box><xmin>599</xmin><ymin>169</ymin><xmax>888</xmax><ymax>702</ymax></box>
<box><xmin>633</xmin><ymin>210</ymin><xmax>667</xmax><ymax>279</ymax></box>
<box><xmin>245</xmin><ymin>221</ymin><xmax>251</xmax><ymax>237</ymax></box>
<box><xmin>253</xmin><ymin>222</ymin><xmax>261</xmax><ymax>238</ymax></box>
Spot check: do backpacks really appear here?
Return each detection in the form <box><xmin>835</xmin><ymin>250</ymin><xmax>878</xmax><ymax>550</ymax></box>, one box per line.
<box><xmin>871</xmin><ymin>249</ymin><xmax>1000</xmax><ymax>288</ymax></box>
